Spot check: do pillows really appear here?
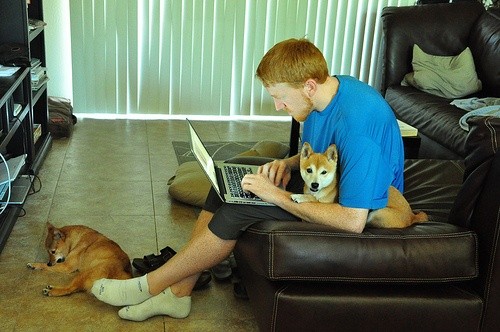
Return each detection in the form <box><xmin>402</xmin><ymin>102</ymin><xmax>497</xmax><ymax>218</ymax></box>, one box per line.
<box><xmin>400</xmin><ymin>44</ymin><xmax>482</xmax><ymax>100</ymax></box>
<box><xmin>167</xmin><ymin>160</ymin><xmax>223</xmax><ymax>206</ymax></box>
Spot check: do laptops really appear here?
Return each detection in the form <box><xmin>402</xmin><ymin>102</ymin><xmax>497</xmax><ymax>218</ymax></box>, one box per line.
<box><xmin>186</xmin><ymin>117</ymin><xmax>286</xmax><ymax>207</ymax></box>
<box><xmin>0</xmin><ymin>154</ymin><xmax>34</xmax><ymax>205</ymax></box>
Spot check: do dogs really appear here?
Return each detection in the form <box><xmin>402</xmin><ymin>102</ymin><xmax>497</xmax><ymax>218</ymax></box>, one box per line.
<box><xmin>288</xmin><ymin>141</ymin><xmax>429</xmax><ymax>230</ymax></box>
<box><xmin>25</xmin><ymin>220</ymin><xmax>134</xmax><ymax>298</ymax></box>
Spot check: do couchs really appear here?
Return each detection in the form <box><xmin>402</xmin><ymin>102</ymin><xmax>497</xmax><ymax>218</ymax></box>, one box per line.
<box><xmin>234</xmin><ymin>0</ymin><xmax>500</xmax><ymax>332</ymax></box>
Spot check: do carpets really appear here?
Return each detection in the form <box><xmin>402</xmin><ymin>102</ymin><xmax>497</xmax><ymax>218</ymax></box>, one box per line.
<box><xmin>173</xmin><ymin>141</ymin><xmax>301</xmax><ymax>222</ymax></box>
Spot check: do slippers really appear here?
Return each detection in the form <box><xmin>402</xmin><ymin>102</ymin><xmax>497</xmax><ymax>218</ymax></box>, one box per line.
<box><xmin>214</xmin><ymin>253</ymin><xmax>233</xmax><ymax>282</ymax></box>
<box><xmin>133</xmin><ymin>246</ymin><xmax>211</xmax><ymax>290</ymax></box>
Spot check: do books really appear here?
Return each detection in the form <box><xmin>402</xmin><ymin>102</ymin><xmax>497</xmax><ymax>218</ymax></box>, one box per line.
<box><xmin>30</xmin><ymin>58</ymin><xmax>49</xmax><ymax>91</ymax></box>
<box><xmin>396</xmin><ymin>118</ymin><xmax>418</xmax><ymax>137</ymax></box>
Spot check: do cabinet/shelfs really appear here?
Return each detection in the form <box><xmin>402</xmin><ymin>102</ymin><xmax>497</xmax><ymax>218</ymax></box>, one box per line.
<box><xmin>0</xmin><ymin>0</ymin><xmax>54</xmax><ymax>255</ymax></box>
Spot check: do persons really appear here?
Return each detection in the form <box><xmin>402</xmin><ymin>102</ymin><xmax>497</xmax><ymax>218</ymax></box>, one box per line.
<box><xmin>92</xmin><ymin>37</ymin><xmax>403</xmax><ymax>321</ymax></box>
